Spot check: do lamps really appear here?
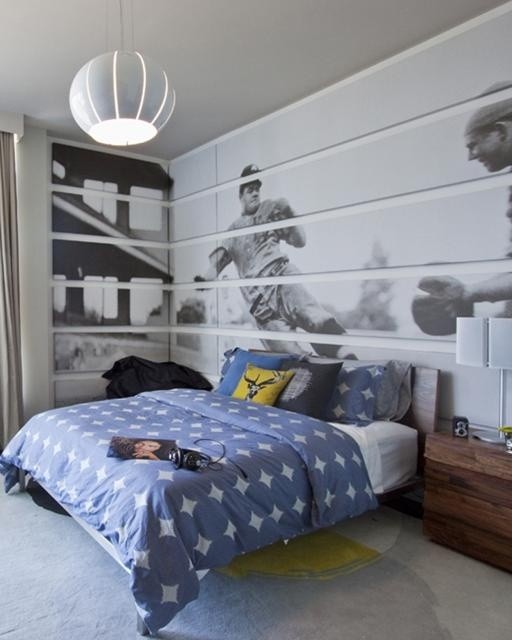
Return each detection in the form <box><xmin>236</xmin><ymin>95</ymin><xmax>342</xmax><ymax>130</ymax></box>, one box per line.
<box><xmin>67</xmin><ymin>0</ymin><xmax>177</xmax><ymax>148</ymax></box>
<box><xmin>455</xmin><ymin>316</ymin><xmax>512</xmax><ymax>445</ymax></box>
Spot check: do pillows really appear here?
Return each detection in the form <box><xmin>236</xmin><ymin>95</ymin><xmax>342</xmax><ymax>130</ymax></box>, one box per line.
<box><xmin>212</xmin><ymin>346</ymin><xmax>413</xmax><ymax>428</ymax></box>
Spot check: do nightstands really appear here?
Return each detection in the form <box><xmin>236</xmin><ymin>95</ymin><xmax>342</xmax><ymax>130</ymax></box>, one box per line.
<box><xmin>423</xmin><ymin>431</ymin><xmax>512</xmax><ymax>575</ymax></box>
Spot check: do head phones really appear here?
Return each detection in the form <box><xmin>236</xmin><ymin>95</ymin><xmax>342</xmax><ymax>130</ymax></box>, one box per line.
<box><xmin>167</xmin><ymin>447</ymin><xmax>213</xmax><ymax>471</ymax></box>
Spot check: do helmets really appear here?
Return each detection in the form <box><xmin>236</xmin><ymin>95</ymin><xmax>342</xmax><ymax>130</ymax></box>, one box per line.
<box><xmin>239</xmin><ymin>164</ymin><xmax>262</xmax><ymax>194</ymax></box>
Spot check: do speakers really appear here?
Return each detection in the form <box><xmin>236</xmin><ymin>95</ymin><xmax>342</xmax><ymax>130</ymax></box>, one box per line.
<box><xmin>452</xmin><ymin>415</ymin><xmax>470</xmax><ymax>439</ymax></box>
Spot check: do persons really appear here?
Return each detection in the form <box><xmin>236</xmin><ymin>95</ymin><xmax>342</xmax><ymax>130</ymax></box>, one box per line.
<box><xmin>441</xmin><ymin>81</ymin><xmax>511</xmax><ymax>318</ymax></box>
<box><xmin>194</xmin><ymin>165</ymin><xmax>358</xmax><ymax>358</ymax></box>
<box><xmin>111</xmin><ymin>435</ymin><xmax>175</xmax><ymax>460</ymax></box>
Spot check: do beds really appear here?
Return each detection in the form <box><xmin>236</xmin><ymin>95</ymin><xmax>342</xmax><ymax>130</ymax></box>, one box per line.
<box><xmin>0</xmin><ymin>347</ymin><xmax>442</xmax><ymax>636</ymax></box>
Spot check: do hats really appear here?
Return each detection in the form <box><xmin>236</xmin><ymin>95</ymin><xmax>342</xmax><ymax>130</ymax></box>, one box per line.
<box><xmin>465</xmin><ymin>82</ymin><xmax>512</xmax><ymax>135</ymax></box>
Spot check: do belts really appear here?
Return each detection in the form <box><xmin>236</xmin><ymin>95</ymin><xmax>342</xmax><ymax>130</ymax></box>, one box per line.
<box><xmin>249</xmin><ymin>257</ymin><xmax>289</xmax><ymax>312</ymax></box>
